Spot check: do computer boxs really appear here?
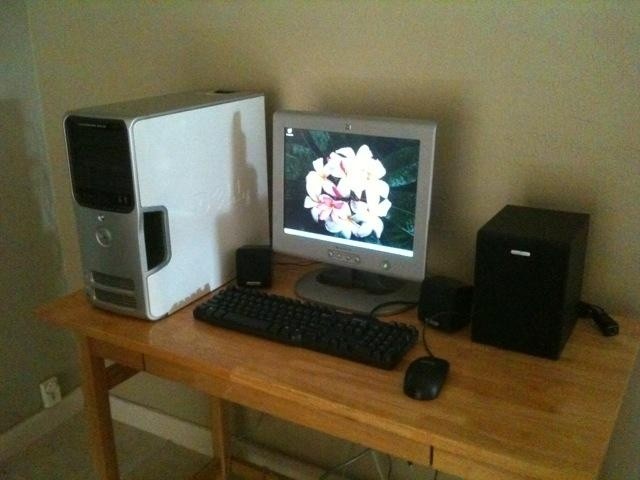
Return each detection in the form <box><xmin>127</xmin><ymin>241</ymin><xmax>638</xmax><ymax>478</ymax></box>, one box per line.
<box><xmin>62</xmin><ymin>87</ymin><xmax>272</xmax><ymax>322</ymax></box>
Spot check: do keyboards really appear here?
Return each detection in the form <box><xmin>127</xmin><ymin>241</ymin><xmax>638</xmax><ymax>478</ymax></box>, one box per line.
<box><xmin>193</xmin><ymin>284</ymin><xmax>421</xmax><ymax>370</ymax></box>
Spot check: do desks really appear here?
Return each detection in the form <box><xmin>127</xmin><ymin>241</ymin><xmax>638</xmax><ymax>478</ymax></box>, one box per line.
<box><xmin>32</xmin><ymin>259</ymin><xmax>640</xmax><ymax>480</ymax></box>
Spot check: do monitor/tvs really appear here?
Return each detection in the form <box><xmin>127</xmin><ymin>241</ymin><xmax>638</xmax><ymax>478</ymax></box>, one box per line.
<box><xmin>270</xmin><ymin>110</ymin><xmax>438</xmax><ymax>316</ymax></box>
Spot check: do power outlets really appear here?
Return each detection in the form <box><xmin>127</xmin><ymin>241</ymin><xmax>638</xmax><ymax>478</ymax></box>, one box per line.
<box><xmin>39</xmin><ymin>375</ymin><xmax>62</xmax><ymax>409</ymax></box>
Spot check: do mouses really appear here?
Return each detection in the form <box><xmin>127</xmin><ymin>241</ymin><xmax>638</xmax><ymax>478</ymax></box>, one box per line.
<box><xmin>403</xmin><ymin>356</ymin><xmax>449</xmax><ymax>400</ymax></box>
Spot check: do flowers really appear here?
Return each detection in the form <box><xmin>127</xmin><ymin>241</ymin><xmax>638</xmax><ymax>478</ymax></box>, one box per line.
<box><xmin>284</xmin><ymin>128</ymin><xmax>419</xmax><ymax>255</ymax></box>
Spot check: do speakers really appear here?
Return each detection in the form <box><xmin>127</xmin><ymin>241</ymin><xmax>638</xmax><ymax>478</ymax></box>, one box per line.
<box><xmin>235</xmin><ymin>245</ymin><xmax>274</xmax><ymax>289</ymax></box>
<box><xmin>470</xmin><ymin>204</ymin><xmax>591</xmax><ymax>360</ymax></box>
<box><xmin>417</xmin><ymin>275</ymin><xmax>473</xmax><ymax>335</ymax></box>
